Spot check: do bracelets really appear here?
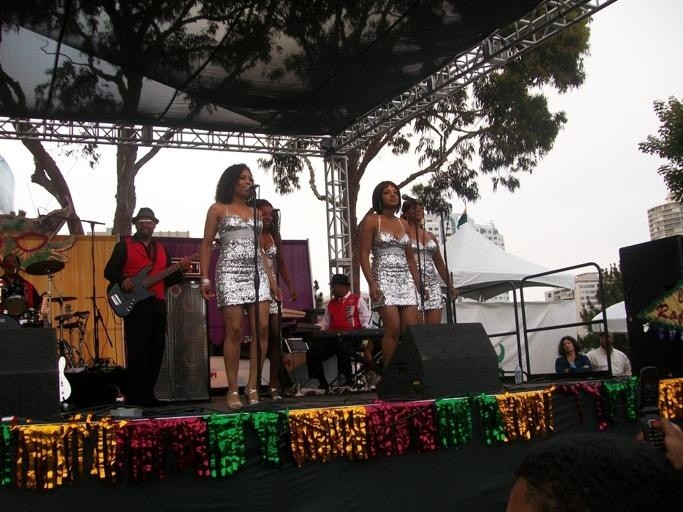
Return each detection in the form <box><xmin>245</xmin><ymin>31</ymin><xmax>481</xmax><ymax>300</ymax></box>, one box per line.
<box><xmin>200</xmin><ymin>275</ymin><xmax>211</xmax><ymax>286</ymax></box>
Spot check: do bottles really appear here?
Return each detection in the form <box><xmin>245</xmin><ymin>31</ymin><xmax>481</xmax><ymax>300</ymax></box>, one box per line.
<box><xmin>514</xmin><ymin>363</ymin><xmax>521</xmax><ymax>384</ymax></box>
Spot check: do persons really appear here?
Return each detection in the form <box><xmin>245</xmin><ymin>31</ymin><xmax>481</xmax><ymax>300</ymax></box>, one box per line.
<box><xmin>395</xmin><ymin>194</ymin><xmax>460</xmax><ymax>324</ymax></box>
<box><xmin>100</xmin><ymin>206</ymin><xmax>200</xmax><ymax>410</ymax></box>
<box><xmin>0</xmin><ymin>253</ymin><xmax>42</xmax><ymax>316</ymax></box>
<box><xmin>356</xmin><ymin>181</ymin><xmax>430</xmax><ymax>371</ymax></box>
<box><xmin>554</xmin><ymin>335</ymin><xmax>591</xmax><ymax>374</ymax></box>
<box><xmin>638</xmin><ymin>417</ymin><xmax>683</xmax><ymax>469</ymax></box>
<box><xmin>502</xmin><ymin>426</ymin><xmax>682</xmax><ymax>511</ymax></box>
<box><xmin>305</xmin><ymin>274</ymin><xmax>374</xmax><ymax>395</ymax></box>
<box><xmin>197</xmin><ymin>164</ymin><xmax>283</xmax><ymax>411</ymax></box>
<box><xmin>583</xmin><ymin>331</ymin><xmax>633</xmax><ymax>376</ymax></box>
<box><xmin>241</xmin><ymin>199</ymin><xmax>300</xmax><ymax>404</ymax></box>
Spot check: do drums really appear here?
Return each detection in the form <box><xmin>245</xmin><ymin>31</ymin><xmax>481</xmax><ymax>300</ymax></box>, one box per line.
<box><xmin>0</xmin><ymin>273</ymin><xmax>28</xmax><ymax>318</ymax></box>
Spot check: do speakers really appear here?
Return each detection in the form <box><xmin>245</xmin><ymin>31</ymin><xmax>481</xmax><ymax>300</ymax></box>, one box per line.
<box><xmin>377</xmin><ymin>323</ymin><xmax>499</xmax><ymax>400</ymax></box>
<box><xmin>124</xmin><ymin>280</ymin><xmax>211</xmax><ymax>404</ymax></box>
<box><xmin>0</xmin><ymin>329</ymin><xmax>59</xmax><ymax>419</ymax></box>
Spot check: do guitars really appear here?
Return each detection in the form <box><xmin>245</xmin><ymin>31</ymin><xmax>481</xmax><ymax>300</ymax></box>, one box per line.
<box><xmin>58</xmin><ymin>355</ymin><xmax>72</xmax><ymax>402</ymax></box>
<box><xmin>107</xmin><ymin>242</ymin><xmax>217</xmax><ymax>317</ymax></box>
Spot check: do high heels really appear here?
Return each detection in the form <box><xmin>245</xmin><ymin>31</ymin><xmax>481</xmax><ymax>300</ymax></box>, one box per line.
<box><xmin>226</xmin><ymin>390</ymin><xmax>242</xmax><ymax>410</ymax></box>
<box><xmin>268</xmin><ymin>386</ymin><xmax>282</xmax><ymax>401</ymax></box>
<box><xmin>244</xmin><ymin>388</ymin><xmax>261</xmax><ymax>409</ymax></box>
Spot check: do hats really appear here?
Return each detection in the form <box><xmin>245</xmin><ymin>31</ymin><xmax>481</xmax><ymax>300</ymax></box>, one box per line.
<box><xmin>131</xmin><ymin>208</ymin><xmax>159</xmax><ymax>224</ymax></box>
<box><xmin>328</xmin><ymin>274</ymin><xmax>351</xmax><ymax>286</ymax></box>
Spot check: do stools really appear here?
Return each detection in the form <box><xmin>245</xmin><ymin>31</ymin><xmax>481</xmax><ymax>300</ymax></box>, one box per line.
<box><xmin>347</xmin><ymin>342</ymin><xmax>372</xmax><ymax>392</ymax></box>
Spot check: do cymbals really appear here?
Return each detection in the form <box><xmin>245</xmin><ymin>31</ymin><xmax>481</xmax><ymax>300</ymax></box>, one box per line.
<box><xmin>55</xmin><ymin>314</ymin><xmax>72</xmax><ymax>320</ymax></box>
<box><xmin>26</xmin><ymin>260</ymin><xmax>65</xmax><ymax>275</ymax></box>
<box><xmin>57</xmin><ymin>322</ymin><xmax>80</xmax><ymax>328</ymax></box>
<box><xmin>47</xmin><ymin>297</ymin><xmax>76</xmax><ymax>302</ymax></box>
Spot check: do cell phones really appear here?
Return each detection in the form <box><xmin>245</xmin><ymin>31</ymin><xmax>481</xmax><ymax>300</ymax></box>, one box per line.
<box><xmin>639</xmin><ymin>365</ymin><xmax>666</xmax><ymax>455</ymax></box>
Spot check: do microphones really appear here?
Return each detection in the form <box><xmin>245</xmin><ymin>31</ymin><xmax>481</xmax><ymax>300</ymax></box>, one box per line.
<box><xmin>246</xmin><ymin>184</ymin><xmax>259</xmax><ymax>190</ymax></box>
<box><xmin>402</xmin><ymin>194</ymin><xmax>419</xmax><ymax>206</ymax></box>
